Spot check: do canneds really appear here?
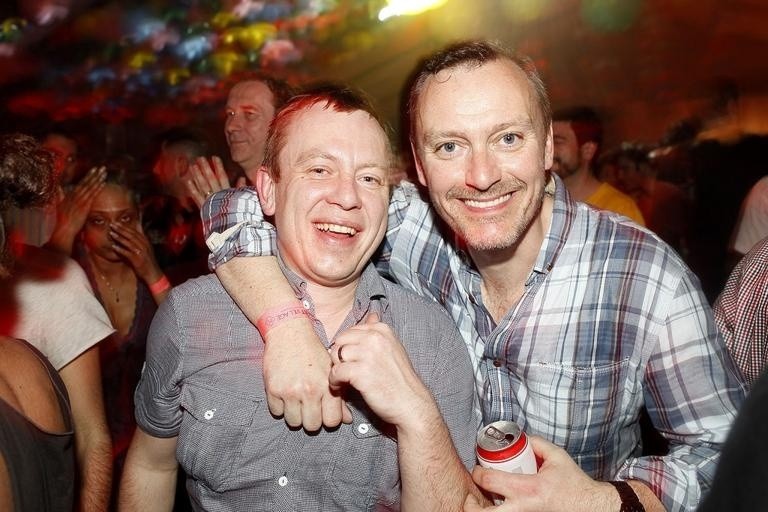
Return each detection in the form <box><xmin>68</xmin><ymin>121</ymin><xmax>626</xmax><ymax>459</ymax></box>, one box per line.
<box><xmin>475</xmin><ymin>419</ymin><xmax>539</xmax><ymax>506</ymax></box>
